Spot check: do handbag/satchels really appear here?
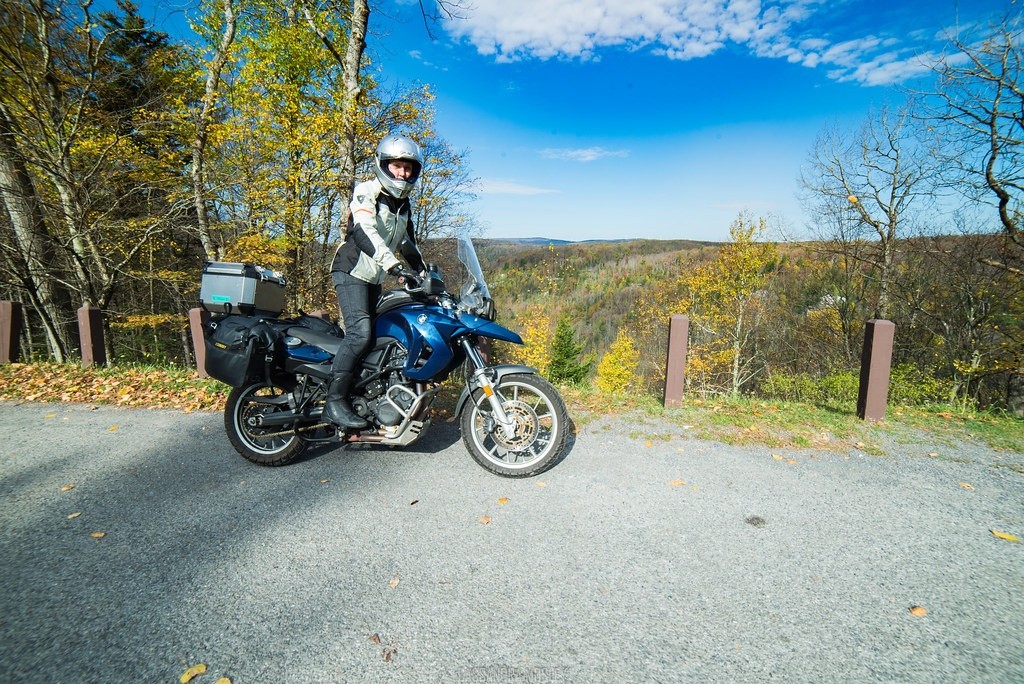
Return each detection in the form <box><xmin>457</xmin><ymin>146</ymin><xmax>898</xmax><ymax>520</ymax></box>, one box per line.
<box><xmin>285</xmin><ymin>313</ymin><xmax>346</xmax><ymax>342</ymax></box>
<box><xmin>204</xmin><ymin>312</ymin><xmax>272</xmax><ymax>388</ymax></box>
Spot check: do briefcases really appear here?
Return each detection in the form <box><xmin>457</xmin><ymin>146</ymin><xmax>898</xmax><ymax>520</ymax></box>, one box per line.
<box><xmin>198</xmin><ymin>261</ymin><xmax>285</xmax><ymax>318</ymax></box>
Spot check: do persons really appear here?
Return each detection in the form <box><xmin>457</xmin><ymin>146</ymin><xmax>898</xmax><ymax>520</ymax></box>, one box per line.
<box><xmin>321</xmin><ymin>133</ymin><xmax>434</xmax><ymax>429</ymax></box>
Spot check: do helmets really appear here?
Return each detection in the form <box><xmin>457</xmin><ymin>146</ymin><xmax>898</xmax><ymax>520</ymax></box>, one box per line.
<box><xmin>375</xmin><ymin>135</ymin><xmax>424</xmax><ymax>200</ymax></box>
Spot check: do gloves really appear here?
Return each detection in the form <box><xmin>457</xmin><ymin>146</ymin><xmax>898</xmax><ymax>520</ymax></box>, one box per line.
<box><xmin>389</xmin><ymin>264</ymin><xmax>423</xmax><ymax>286</ymax></box>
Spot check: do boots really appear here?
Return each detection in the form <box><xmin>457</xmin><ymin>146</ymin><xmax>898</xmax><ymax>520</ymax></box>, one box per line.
<box><xmin>321</xmin><ymin>371</ymin><xmax>368</xmax><ymax>428</ymax></box>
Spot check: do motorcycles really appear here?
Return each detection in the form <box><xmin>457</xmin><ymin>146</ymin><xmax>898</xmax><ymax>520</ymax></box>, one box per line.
<box><xmin>198</xmin><ymin>224</ymin><xmax>569</xmax><ymax>481</ymax></box>
